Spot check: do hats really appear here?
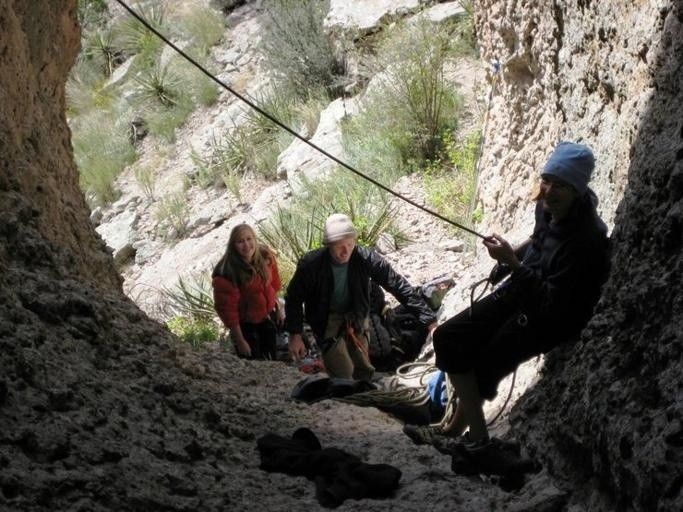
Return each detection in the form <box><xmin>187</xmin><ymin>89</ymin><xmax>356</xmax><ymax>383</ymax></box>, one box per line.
<box><xmin>541</xmin><ymin>141</ymin><xmax>595</xmax><ymax>198</ymax></box>
<box><xmin>322</xmin><ymin>213</ymin><xmax>357</xmax><ymax>246</ymax></box>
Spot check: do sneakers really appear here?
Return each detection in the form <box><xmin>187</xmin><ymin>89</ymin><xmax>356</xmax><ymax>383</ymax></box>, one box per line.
<box><xmin>403</xmin><ymin>424</ymin><xmax>495</xmax><ymax>459</ymax></box>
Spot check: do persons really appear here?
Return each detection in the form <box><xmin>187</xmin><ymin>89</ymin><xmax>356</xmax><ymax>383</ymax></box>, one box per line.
<box><xmin>211</xmin><ymin>224</ymin><xmax>285</xmax><ymax>359</ymax></box>
<box><xmin>402</xmin><ymin>142</ymin><xmax>609</xmax><ymax>456</ymax></box>
<box><xmin>285</xmin><ymin>214</ymin><xmax>440</xmax><ymax>379</ymax></box>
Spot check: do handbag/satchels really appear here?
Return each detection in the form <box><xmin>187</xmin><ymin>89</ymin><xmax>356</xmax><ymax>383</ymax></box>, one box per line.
<box><xmin>275</xmin><ymin>296</ymin><xmax>286</xmax><ymax>320</ymax></box>
